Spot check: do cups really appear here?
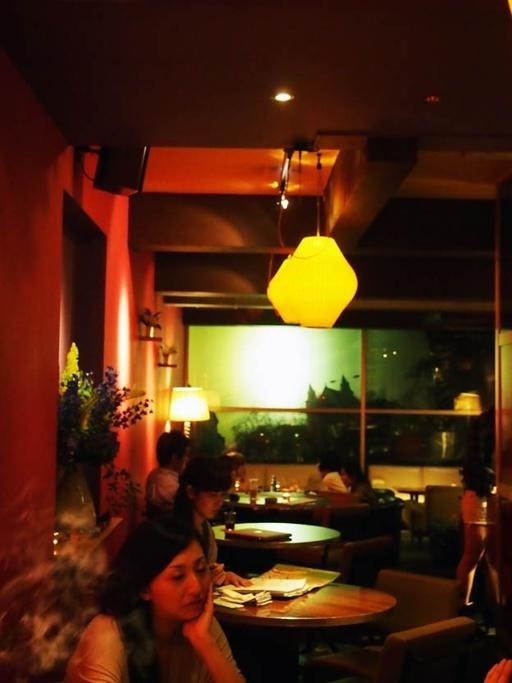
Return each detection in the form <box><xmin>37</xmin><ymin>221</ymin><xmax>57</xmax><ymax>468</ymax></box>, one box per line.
<box><xmin>250</xmin><ymin>478</ymin><xmax>258</xmax><ymax>503</ymax></box>
<box><xmin>225</xmin><ymin>511</ymin><xmax>235</xmax><ymax>530</ymax></box>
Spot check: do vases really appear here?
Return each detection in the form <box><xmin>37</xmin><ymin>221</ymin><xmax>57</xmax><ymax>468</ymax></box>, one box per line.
<box><xmin>59</xmin><ymin>464</ymin><xmax>96</xmax><ymax>534</ymax></box>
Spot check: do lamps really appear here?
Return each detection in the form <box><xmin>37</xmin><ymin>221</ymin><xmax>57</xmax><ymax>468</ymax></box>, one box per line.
<box><xmin>168</xmin><ymin>387</ymin><xmax>211</xmax><ymax>438</ymax></box>
<box><xmin>267</xmin><ymin>149</ymin><xmax>301</xmax><ymax>324</ymax></box>
<box><xmin>454</xmin><ymin>392</ymin><xmax>482</xmax><ymax>426</ymax></box>
<box><xmin>281</xmin><ymin>150</ymin><xmax>358</xmax><ymax>328</ymax></box>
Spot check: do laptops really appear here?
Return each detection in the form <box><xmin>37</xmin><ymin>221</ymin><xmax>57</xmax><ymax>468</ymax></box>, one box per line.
<box><xmin>226</xmin><ymin>529</ymin><xmax>292</xmax><ymax>540</ymax></box>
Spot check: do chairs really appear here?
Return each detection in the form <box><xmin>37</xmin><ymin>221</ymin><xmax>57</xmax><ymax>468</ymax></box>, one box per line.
<box><xmin>311</xmin><ymin>616</ymin><xmax>475</xmax><ymax>682</ymax></box>
<box><xmin>321</xmin><ymin>569</ymin><xmax>463</xmax><ymax>652</ymax></box>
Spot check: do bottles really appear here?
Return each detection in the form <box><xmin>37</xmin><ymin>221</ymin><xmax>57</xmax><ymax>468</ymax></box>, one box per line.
<box><xmin>270</xmin><ymin>475</ymin><xmax>276</xmax><ymax>490</ymax></box>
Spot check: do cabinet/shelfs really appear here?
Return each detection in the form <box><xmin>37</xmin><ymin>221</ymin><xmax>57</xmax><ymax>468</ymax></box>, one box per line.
<box><xmin>139</xmin><ymin>336</ymin><xmax>178</xmax><ymax>367</ymax></box>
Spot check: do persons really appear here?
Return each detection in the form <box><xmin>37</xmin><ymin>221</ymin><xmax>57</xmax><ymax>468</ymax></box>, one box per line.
<box><xmin>156</xmin><ymin>458</ymin><xmax>249</xmax><ymax>586</ymax></box>
<box><xmin>303</xmin><ymin>450</ymin><xmax>371</xmax><ymax>525</ymax></box>
<box><xmin>483</xmin><ymin>658</ymin><xmax>512</xmax><ymax>682</ymax></box>
<box><xmin>67</xmin><ymin>518</ymin><xmax>246</xmax><ymax>683</ymax></box>
<box><xmin>452</xmin><ymin>405</ymin><xmax>507</xmax><ymax>615</ymax></box>
<box><xmin>146</xmin><ymin>430</ymin><xmax>191</xmax><ymax>511</ymax></box>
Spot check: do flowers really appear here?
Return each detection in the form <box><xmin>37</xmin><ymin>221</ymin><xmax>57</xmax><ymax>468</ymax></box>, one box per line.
<box><xmin>60</xmin><ymin>341</ymin><xmax>154</xmax><ymax>508</ymax></box>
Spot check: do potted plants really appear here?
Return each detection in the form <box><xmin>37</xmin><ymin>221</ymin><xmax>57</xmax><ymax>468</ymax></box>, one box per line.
<box><xmin>159</xmin><ymin>343</ymin><xmax>179</xmax><ymax>365</ymax></box>
<box><xmin>139</xmin><ymin>308</ymin><xmax>162</xmax><ymax>338</ymax></box>
